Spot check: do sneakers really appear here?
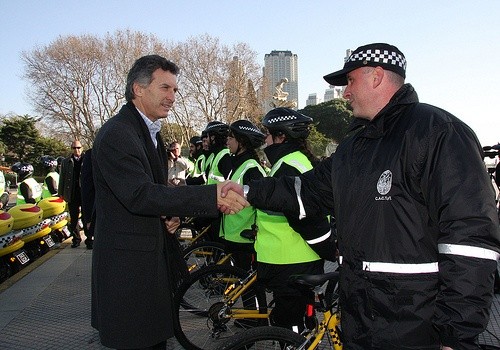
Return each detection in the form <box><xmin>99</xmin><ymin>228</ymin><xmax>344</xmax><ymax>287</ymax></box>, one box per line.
<box><xmin>194</xmin><ymin>249</ymin><xmax>270</xmax><ymax>328</ymax></box>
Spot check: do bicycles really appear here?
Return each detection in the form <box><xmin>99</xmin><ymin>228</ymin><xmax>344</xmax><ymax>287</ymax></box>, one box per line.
<box><xmin>170</xmin><ymin>213</ymin><xmax>348</xmax><ymax>350</ymax></box>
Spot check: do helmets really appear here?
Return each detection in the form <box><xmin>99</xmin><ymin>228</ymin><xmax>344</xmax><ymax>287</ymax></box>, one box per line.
<box><xmin>191</xmin><ymin>136</ymin><xmax>204</xmax><ymax>144</ymax></box>
<box><xmin>56</xmin><ymin>156</ymin><xmax>65</xmax><ymax>166</ymax></box>
<box><xmin>42</xmin><ymin>158</ymin><xmax>58</xmax><ymax>172</ymax></box>
<box><xmin>19</xmin><ymin>163</ymin><xmax>34</xmax><ymax>180</ymax></box>
<box><xmin>204</xmin><ymin>121</ymin><xmax>230</xmax><ymax>134</ymax></box>
<box><xmin>230</xmin><ymin>120</ymin><xmax>268</xmax><ymax>146</ymax></box>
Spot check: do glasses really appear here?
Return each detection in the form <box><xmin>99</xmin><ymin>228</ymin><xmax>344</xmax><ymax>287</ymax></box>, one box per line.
<box><xmin>261</xmin><ymin>107</ymin><xmax>313</xmax><ymax>138</ymax></box>
<box><xmin>71</xmin><ymin>146</ymin><xmax>82</xmax><ymax>149</ymax></box>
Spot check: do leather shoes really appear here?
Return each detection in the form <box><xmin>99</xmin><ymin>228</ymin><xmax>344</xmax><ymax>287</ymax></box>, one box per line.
<box><xmin>71</xmin><ymin>240</ymin><xmax>82</xmax><ymax>247</ymax></box>
<box><xmin>87</xmin><ymin>240</ymin><xmax>92</xmax><ymax>249</ymax></box>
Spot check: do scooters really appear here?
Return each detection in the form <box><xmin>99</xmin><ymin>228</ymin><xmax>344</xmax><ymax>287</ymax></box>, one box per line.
<box><xmin>0</xmin><ymin>192</ymin><xmax>83</xmax><ymax>273</ymax></box>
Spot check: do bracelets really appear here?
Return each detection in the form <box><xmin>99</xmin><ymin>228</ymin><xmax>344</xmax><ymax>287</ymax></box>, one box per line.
<box><xmin>243</xmin><ymin>185</ymin><xmax>250</xmax><ymax>201</ymax></box>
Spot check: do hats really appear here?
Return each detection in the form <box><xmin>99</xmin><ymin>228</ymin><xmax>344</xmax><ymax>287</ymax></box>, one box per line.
<box><xmin>323</xmin><ymin>44</ymin><xmax>407</xmax><ymax>87</ymax></box>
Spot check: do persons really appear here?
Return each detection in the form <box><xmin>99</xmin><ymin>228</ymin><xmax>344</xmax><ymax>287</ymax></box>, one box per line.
<box><xmin>168</xmin><ymin>121</ymin><xmax>231</xmax><ymax>280</ymax></box>
<box><xmin>40</xmin><ymin>155</ymin><xmax>60</xmax><ymax>199</ymax></box>
<box><xmin>217</xmin><ymin>43</ymin><xmax>500</xmax><ymax>350</ymax></box>
<box><xmin>57</xmin><ymin>140</ymin><xmax>93</xmax><ymax>251</ymax></box>
<box><xmin>218</xmin><ymin>120</ymin><xmax>269</xmax><ymax>330</ymax></box>
<box><xmin>91</xmin><ymin>54</ymin><xmax>251</xmax><ymax>350</ymax></box>
<box><xmin>253</xmin><ymin>107</ymin><xmax>340</xmax><ymax>349</ymax></box>
<box><xmin>11</xmin><ymin>161</ymin><xmax>42</xmax><ymax>205</ymax></box>
<box><xmin>0</xmin><ymin>169</ymin><xmax>11</xmax><ymax>212</ymax></box>
